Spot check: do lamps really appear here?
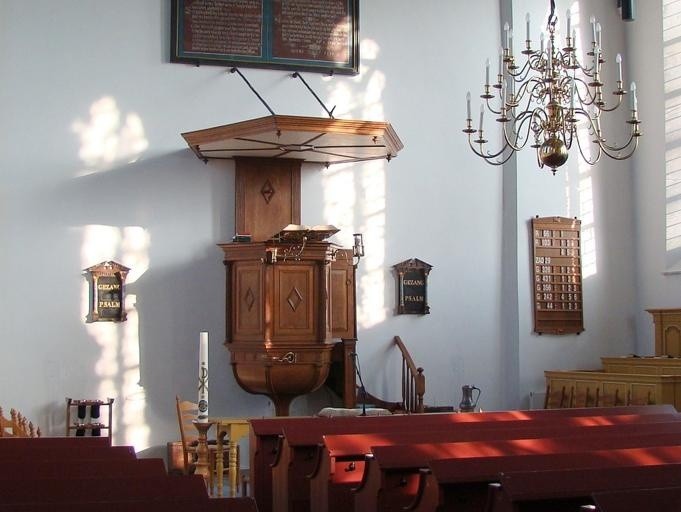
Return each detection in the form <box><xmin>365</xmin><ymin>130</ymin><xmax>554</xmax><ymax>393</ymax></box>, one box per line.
<box><xmin>462</xmin><ymin>0</ymin><xmax>644</xmax><ymax>175</ymax></box>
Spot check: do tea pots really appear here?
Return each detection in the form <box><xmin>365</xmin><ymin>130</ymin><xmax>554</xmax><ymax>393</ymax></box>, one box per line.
<box><xmin>459</xmin><ymin>384</ymin><xmax>481</xmax><ymax>413</ymax></box>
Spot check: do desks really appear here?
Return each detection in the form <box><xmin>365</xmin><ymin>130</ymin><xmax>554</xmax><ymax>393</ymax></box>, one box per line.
<box><xmin>600</xmin><ymin>354</ymin><xmax>681</xmax><ymax>375</ymax></box>
<box><xmin>644</xmin><ymin>309</ymin><xmax>681</xmax><ymax>356</ymax></box>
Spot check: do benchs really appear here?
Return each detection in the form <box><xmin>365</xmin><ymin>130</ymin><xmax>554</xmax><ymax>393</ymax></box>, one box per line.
<box><xmin>0</xmin><ymin>404</ymin><xmax>681</xmax><ymax>512</ymax></box>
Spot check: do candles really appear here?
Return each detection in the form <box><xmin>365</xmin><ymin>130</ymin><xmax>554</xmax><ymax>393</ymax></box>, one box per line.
<box><xmin>197</xmin><ymin>331</ymin><xmax>209</xmax><ymax>423</ymax></box>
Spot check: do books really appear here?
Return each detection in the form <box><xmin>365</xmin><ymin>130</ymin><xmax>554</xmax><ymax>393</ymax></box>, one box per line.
<box><xmin>619</xmin><ymin>353</ymin><xmax>681</xmax><ymax>359</ymax></box>
<box><xmin>272</xmin><ymin>223</ymin><xmax>342</xmax><ymax>241</ymax></box>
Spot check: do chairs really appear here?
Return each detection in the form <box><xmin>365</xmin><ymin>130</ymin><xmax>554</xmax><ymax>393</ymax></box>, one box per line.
<box><xmin>175</xmin><ymin>394</ymin><xmax>241</xmax><ymax>498</ymax></box>
